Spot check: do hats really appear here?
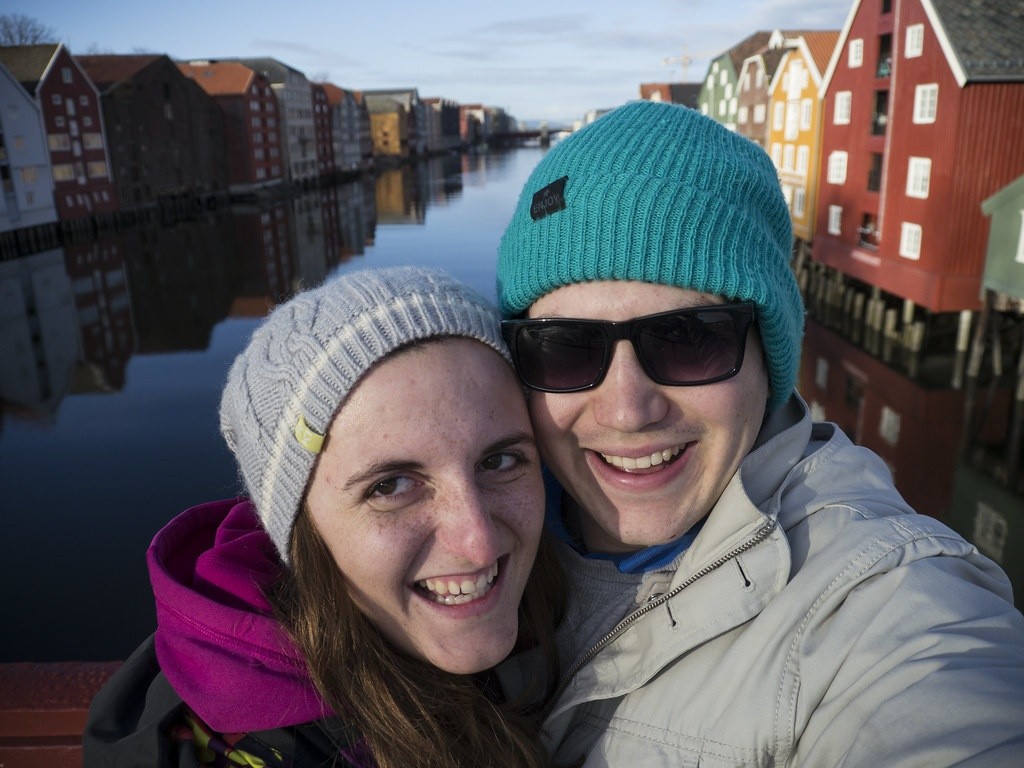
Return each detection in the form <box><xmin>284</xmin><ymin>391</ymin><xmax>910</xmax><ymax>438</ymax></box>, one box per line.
<box><xmin>219</xmin><ymin>266</ymin><xmax>519</xmax><ymax>574</ymax></box>
<box><xmin>496</xmin><ymin>100</ymin><xmax>805</xmax><ymax>414</ymax></box>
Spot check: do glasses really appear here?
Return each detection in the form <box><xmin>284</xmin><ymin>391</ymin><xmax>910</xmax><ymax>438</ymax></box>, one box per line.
<box><xmin>502</xmin><ymin>301</ymin><xmax>757</xmax><ymax>393</ymax></box>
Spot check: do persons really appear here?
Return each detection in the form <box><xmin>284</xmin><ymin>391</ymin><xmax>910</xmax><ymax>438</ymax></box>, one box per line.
<box><xmin>476</xmin><ymin>100</ymin><xmax>1024</xmax><ymax>768</ymax></box>
<box><xmin>82</xmin><ymin>264</ymin><xmax>546</xmax><ymax>768</ymax></box>
